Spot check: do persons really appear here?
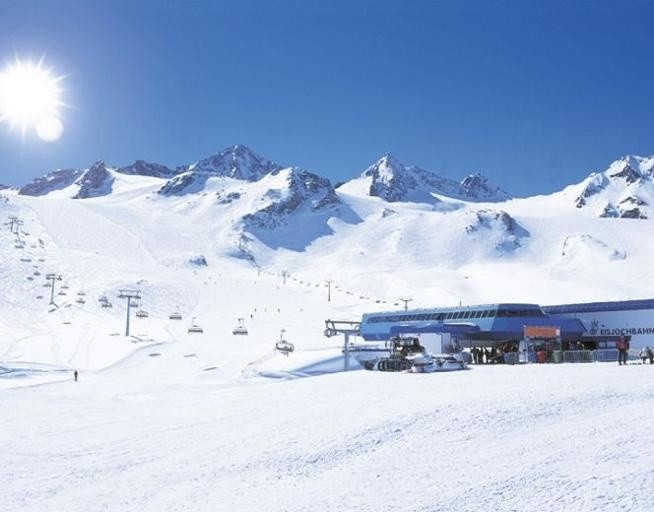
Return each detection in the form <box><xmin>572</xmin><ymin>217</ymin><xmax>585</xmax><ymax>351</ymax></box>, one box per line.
<box><xmin>639</xmin><ymin>347</ymin><xmax>654</xmax><ymax>364</ymax></box>
<box><xmin>471</xmin><ymin>343</ymin><xmax>518</xmax><ymax>364</ymax></box>
<box><xmin>615</xmin><ymin>333</ymin><xmax>629</xmax><ymax>365</ymax></box>
